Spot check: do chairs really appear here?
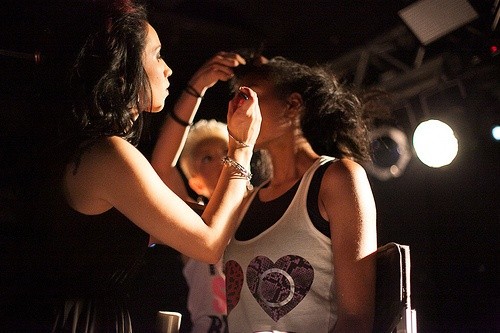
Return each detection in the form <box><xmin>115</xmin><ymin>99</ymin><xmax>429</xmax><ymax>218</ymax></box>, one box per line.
<box><xmin>372</xmin><ymin>242</ymin><xmax>412</xmax><ymax>333</ymax></box>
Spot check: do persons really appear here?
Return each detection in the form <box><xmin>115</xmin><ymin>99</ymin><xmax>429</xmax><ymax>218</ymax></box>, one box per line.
<box><xmin>225</xmin><ymin>54</ymin><xmax>377</xmax><ymax>333</ymax></box>
<box><xmin>0</xmin><ymin>1</ymin><xmax>262</xmax><ymax>333</ymax></box>
<box><xmin>152</xmin><ymin>48</ymin><xmax>256</xmax><ymax>333</ymax></box>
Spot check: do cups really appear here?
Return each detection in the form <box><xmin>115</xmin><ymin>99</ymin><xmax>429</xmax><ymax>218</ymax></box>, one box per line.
<box><xmin>156</xmin><ymin>310</ymin><xmax>181</xmax><ymax>333</ymax></box>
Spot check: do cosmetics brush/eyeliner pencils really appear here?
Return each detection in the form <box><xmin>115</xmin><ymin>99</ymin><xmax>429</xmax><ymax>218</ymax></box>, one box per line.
<box><xmin>233</xmin><ymin>87</ymin><xmax>250</xmax><ymax>102</ymax></box>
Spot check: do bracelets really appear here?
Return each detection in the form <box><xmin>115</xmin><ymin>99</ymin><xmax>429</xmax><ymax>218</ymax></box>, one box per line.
<box><xmin>220</xmin><ymin>154</ymin><xmax>252</xmax><ymax>182</ymax></box>
<box><xmin>182</xmin><ymin>82</ymin><xmax>205</xmax><ymax>102</ymax></box>
<box><xmin>168</xmin><ymin>109</ymin><xmax>194</xmax><ymax>129</ymax></box>
